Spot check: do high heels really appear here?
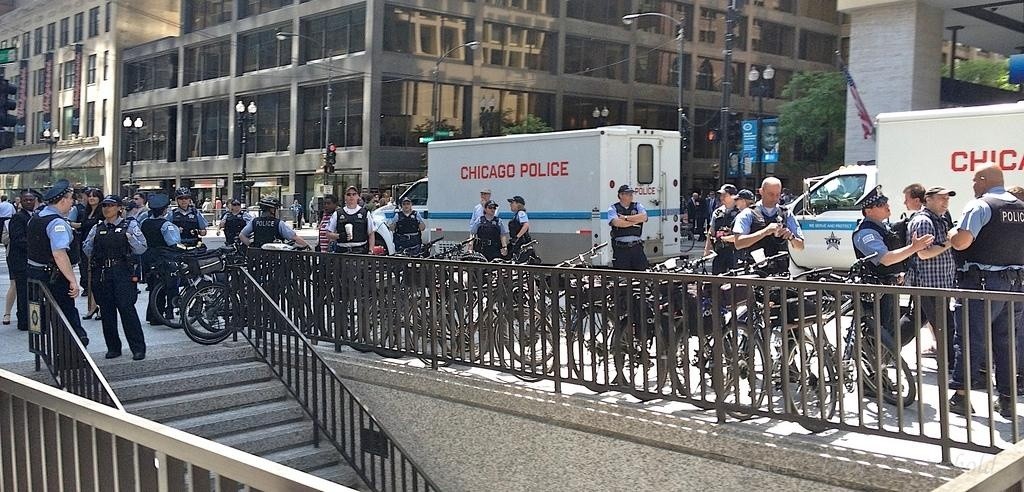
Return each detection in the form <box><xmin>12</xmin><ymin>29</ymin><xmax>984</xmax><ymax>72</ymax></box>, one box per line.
<box><xmin>83</xmin><ymin>306</ymin><xmax>102</xmax><ymax>320</ymax></box>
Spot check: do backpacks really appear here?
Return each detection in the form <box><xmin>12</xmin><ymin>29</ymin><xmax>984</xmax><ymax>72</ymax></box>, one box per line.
<box><xmin>888</xmin><ymin>211</ymin><xmax>938</xmax><ymax>274</ymax></box>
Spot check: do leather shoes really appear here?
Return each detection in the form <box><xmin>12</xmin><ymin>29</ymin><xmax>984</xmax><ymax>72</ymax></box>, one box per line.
<box><xmin>106</xmin><ymin>351</ymin><xmax>121</xmax><ymax>359</ymax></box>
<box><xmin>3</xmin><ymin>314</ymin><xmax>10</xmax><ymax>325</ymax></box>
<box><xmin>133</xmin><ymin>352</ymin><xmax>145</xmax><ymax>360</ymax></box>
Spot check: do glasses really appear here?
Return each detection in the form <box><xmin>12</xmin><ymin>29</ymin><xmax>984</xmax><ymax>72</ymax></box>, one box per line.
<box><xmin>233</xmin><ymin>203</ymin><xmax>240</xmax><ymax>206</ymax></box>
<box><xmin>347</xmin><ymin>193</ymin><xmax>356</xmax><ymax>196</ymax></box>
<box><xmin>179</xmin><ymin>197</ymin><xmax>189</xmax><ymax>200</ymax></box>
<box><xmin>101</xmin><ymin>203</ymin><xmax>114</xmax><ymax>207</ymax></box>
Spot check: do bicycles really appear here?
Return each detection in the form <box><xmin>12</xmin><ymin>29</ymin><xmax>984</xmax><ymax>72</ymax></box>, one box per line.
<box><xmin>152</xmin><ymin>234</ymin><xmax>914</xmax><ymax>433</ymax></box>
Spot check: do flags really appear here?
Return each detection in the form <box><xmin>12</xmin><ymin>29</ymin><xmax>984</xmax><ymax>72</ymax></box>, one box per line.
<box><xmin>836</xmin><ymin>59</ymin><xmax>875</xmax><ymax>141</ymax></box>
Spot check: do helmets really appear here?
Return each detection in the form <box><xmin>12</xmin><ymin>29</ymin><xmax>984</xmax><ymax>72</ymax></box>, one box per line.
<box><xmin>174</xmin><ymin>187</ymin><xmax>191</xmax><ymax>198</ymax></box>
<box><xmin>258</xmin><ymin>196</ymin><xmax>281</xmax><ymax>209</ymax></box>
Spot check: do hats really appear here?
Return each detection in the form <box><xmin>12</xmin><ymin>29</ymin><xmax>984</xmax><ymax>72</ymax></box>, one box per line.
<box><xmin>924</xmin><ymin>187</ymin><xmax>956</xmax><ymax>196</ymax></box>
<box><xmin>231</xmin><ymin>198</ymin><xmax>241</xmax><ymax>203</ymax></box>
<box><xmin>618</xmin><ymin>185</ymin><xmax>634</xmax><ymax>192</ymax></box>
<box><xmin>854</xmin><ymin>185</ymin><xmax>888</xmax><ymax>208</ymax></box>
<box><xmin>345</xmin><ymin>185</ymin><xmax>358</xmax><ymax>192</ymax></box>
<box><xmin>507</xmin><ymin>195</ymin><xmax>525</xmax><ymax>205</ymax></box>
<box><xmin>99</xmin><ymin>194</ymin><xmax>122</xmax><ymax>205</ymax></box>
<box><xmin>717</xmin><ymin>183</ymin><xmax>738</xmax><ymax>195</ymax></box>
<box><xmin>402</xmin><ymin>196</ymin><xmax>411</xmax><ymax>202</ymax></box>
<box><xmin>480</xmin><ymin>188</ymin><xmax>499</xmax><ymax>207</ymax></box>
<box><xmin>149</xmin><ymin>193</ymin><xmax>169</xmax><ymax>209</ymax></box>
<box><xmin>20</xmin><ymin>179</ymin><xmax>73</xmax><ymax>201</ymax></box>
<box><xmin>730</xmin><ymin>189</ymin><xmax>754</xmax><ymax>200</ymax></box>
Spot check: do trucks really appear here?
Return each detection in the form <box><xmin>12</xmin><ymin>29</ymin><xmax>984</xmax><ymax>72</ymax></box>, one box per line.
<box><xmin>371</xmin><ymin>120</ymin><xmax>683</xmax><ymax>273</ymax></box>
<box><xmin>779</xmin><ymin>99</ymin><xmax>1023</xmax><ymax>281</ymax></box>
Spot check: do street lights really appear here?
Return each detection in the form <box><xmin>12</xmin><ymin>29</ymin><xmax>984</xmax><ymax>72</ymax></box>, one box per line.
<box><xmin>272</xmin><ymin>27</ymin><xmax>332</xmax><ymax>183</ymax></box>
<box><xmin>621</xmin><ymin>11</ymin><xmax>687</xmax><ymax>138</ymax></box>
<box><xmin>121</xmin><ymin>115</ymin><xmax>144</xmax><ymax>196</ymax></box>
<box><xmin>430</xmin><ymin>38</ymin><xmax>483</xmax><ymax>139</ymax></box>
<box><xmin>234</xmin><ymin>98</ymin><xmax>258</xmax><ymax>203</ymax></box>
<box><xmin>39</xmin><ymin>127</ymin><xmax>61</xmax><ymax>189</ymax></box>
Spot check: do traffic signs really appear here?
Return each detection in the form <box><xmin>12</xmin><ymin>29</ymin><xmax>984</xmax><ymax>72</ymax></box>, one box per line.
<box><xmin>0</xmin><ymin>46</ymin><xmax>18</xmax><ymax>64</ymax></box>
<box><xmin>435</xmin><ymin>131</ymin><xmax>454</xmax><ymax>137</ymax></box>
<box><xmin>418</xmin><ymin>137</ymin><xmax>435</xmax><ymax>145</ymax></box>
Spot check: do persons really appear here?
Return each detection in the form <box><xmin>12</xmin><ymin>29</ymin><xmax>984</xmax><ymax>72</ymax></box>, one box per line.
<box><xmin>239</xmin><ymin>195</ymin><xmax>313</xmax><ymax>251</ymax></box>
<box><xmin>1</xmin><ymin>176</ymin><xmax>207</xmax><ymax>368</ymax></box>
<box><xmin>469</xmin><ymin>188</ymin><xmax>492</xmax><ymax>226</ymax></box>
<box><xmin>201</xmin><ymin>196</ymin><xmax>254</xmax><ymax>263</ymax></box>
<box><xmin>308</xmin><ymin>189</ymin><xmax>394</xmax><ymax>255</ymax></box>
<box><xmin>468</xmin><ymin>199</ymin><xmax>508</xmax><ymax>262</ymax></box>
<box><xmin>289</xmin><ymin>199</ymin><xmax>303</xmax><ymax>229</ymax></box>
<box><xmin>387</xmin><ymin>196</ymin><xmax>426</xmax><ymax>258</ymax></box>
<box><xmin>507</xmin><ymin>195</ymin><xmax>536</xmax><ymax>262</ymax></box>
<box><xmin>850</xmin><ymin>158</ymin><xmax>1024</xmax><ymax>419</ymax></box>
<box><xmin>606</xmin><ymin>183</ymin><xmax>651</xmax><ymax>272</ymax></box>
<box><xmin>687</xmin><ymin>176</ymin><xmax>805</xmax><ymax>276</ymax></box>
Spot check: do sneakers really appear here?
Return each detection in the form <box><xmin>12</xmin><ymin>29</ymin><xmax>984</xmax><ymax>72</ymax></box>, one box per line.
<box><xmin>994</xmin><ymin>394</ymin><xmax>1012</xmax><ymax>417</ymax></box>
<box><xmin>948</xmin><ymin>391</ymin><xmax>966</xmax><ymax>415</ymax></box>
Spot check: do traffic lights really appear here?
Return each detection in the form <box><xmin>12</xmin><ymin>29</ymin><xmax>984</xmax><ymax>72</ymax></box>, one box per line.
<box><xmin>1</xmin><ymin>78</ymin><xmax>18</xmax><ymax>152</ymax></box>
<box><xmin>324</xmin><ymin>142</ymin><xmax>337</xmax><ymax>174</ymax></box>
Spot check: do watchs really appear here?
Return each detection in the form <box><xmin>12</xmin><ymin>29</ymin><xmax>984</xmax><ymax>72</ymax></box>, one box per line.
<box><xmin>624</xmin><ymin>215</ymin><xmax>627</xmax><ymax>221</ymax></box>
